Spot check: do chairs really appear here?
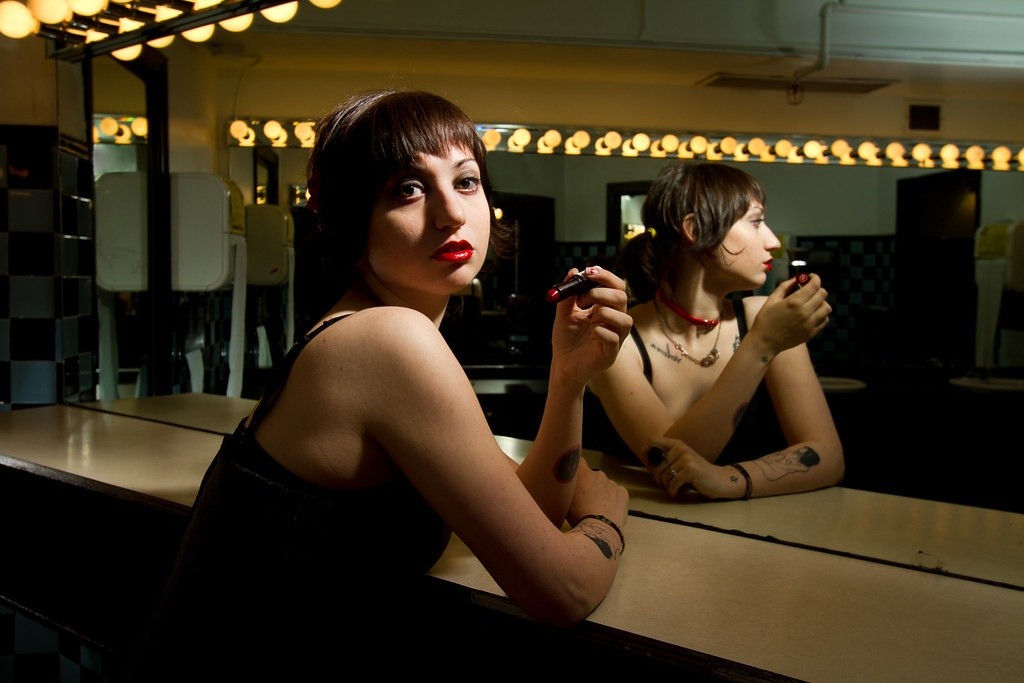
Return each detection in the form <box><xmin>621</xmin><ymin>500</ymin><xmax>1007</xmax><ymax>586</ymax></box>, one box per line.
<box><xmin>448</xmin><ymin>277</ymin><xmax>505</xmax><ymax>356</ymax></box>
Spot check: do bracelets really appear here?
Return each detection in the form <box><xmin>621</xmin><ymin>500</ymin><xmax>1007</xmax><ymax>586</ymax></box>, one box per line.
<box><xmin>727</xmin><ymin>460</ymin><xmax>753</xmax><ymax>499</ymax></box>
<box><xmin>571</xmin><ymin>514</ymin><xmax>626</xmax><ymax>557</ymax></box>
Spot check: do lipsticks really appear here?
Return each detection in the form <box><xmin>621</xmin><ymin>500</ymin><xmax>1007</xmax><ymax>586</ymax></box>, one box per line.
<box><xmin>544</xmin><ymin>266</ymin><xmax>602</xmax><ymax>304</ymax></box>
<box><xmin>791</xmin><ymin>273</ymin><xmax>810</xmax><ymax>288</ymax></box>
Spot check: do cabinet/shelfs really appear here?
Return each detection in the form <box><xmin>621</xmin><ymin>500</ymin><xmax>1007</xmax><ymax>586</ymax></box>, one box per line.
<box><xmin>477</xmin><ymin>189</ymin><xmax>555</xmax><ymax>302</ymax></box>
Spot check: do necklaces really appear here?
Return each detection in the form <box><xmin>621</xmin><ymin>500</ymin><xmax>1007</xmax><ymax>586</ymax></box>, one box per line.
<box><xmin>656</xmin><ymin>286</ymin><xmax>723</xmax><ymax>328</ymax></box>
<box><xmin>651</xmin><ymin>303</ymin><xmax>725</xmax><ymax>366</ymax></box>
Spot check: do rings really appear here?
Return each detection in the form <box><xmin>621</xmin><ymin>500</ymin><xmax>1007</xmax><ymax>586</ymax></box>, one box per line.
<box><xmin>669</xmin><ymin>467</ymin><xmax>679</xmax><ymax>480</ymax></box>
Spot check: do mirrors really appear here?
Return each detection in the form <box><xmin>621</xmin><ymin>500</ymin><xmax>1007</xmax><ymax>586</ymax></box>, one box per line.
<box><xmin>605</xmin><ymin>179</ymin><xmax>653</xmax><ymax>275</ymax></box>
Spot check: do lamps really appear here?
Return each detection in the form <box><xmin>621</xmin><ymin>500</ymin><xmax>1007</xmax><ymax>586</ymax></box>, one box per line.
<box><xmin>650</xmin><ymin>134</ymin><xmax>679</xmax><ymax>158</ymax></box>
<box><xmin>564</xmin><ymin>130</ymin><xmax>591</xmax><ymax>155</ymax></box>
<box><xmin>941</xmin><ymin>145</ymin><xmax>987</xmax><ymax>169</ymax></box>
<box><xmin>59</xmin><ymin>0</ymin><xmax>1024</xmax><ymax>516</ymax></box>
<box><xmin>734</xmin><ymin>137</ymin><xmax>765</xmax><ymax>162</ymax></box>
<box><xmin>294</xmin><ymin>121</ymin><xmax>316</xmax><ymax>148</ymax></box>
<box><xmin>129</xmin><ymin>115</ymin><xmax>148</xmax><ymax>142</ymax></box>
<box><xmin>264</xmin><ymin>120</ymin><xmax>288</xmax><ymax>148</ymax></box>
<box><xmin>788</xmin><ymin>140</ymin><xmax>820</xmax><ymax>163</ymax></box>
<box><xmin>0</xmin><ymin>0</ymin><xmax>59</xmax><ymax>45</ymax></box>
<box><xmin>537</xmin><ymin>129</ymin><xmax>563</xmax><ymax>155</ymax></box>
<box><xmin>622</xmin><ymin>132</ymin><xmax>651</xmax><ymax>157</ymax></box>
<box><xmin>677</xmin><ymin>135</ymin><xmax>708</xmax><ymax>159</ymax></box>
<box><xmin>841</xmin><ymin>140</ymin><xmax>876</xmax><ymax>164</ymax></box>
<box><xmin>110</xmin><ymin>44</ymin><xmax>144</xmax><ymax>62</ymax></box>
<box><xmin>1013</xmin><ymin>146</ymin><xmax>1024</xmax><ymax>170</ymax></box>
<box><xmin>218</xmin><ymin>12</ymin><xmax>254</xmax><ymax>32</ymax></box>
<box><xmin>145</xmin><ymin>35</ymin><xmax>177</xmax><ymax>49</ymax></box>
<box><xmin>309</xmin><ymin>0</ymin><xmax>341</xmax><ymax>8</ymax></box>
<box><xmin>229</xmin><ymin>119</ymin><xmax>256</xmax><ymax>148</ymax></box>
<box><xmin>918</xmin><ymin>143</ymin><xmax>960</xmax><ymax>169</ymax></box>
<box><xmin>260</xmin><ymin>0</ymin><xmax>300</xmax><ymax>24</ymax></box>
<box><xmin>180</xmin><ymin>23</ymin><xmax>216</xmax><ymax>43</ymax></box>
<box><xmin>27</xmin><ymin>0</ymin><xmax>102</xmax><ymax>40</ymax></box>
<box><xmin>507</xmin><ymin>129</ymin><xmax>532</xmax><ymax>154</ymax></box>
<box><xmin>968</xmin><ymin>144</ymin><xmax>1013</xmax><ymax>171</ymax></box>
<box><xmin>70</xmin><ymin>0</ymin><xmax>159</xmax><ymax>23</ymax></box>
<box><xmin>761</xmin><ymin>138</ymin><xmax>792</xmax><ymax>163</ymax></box>
<box><xmin>99</xmin><ymin>116</ymin><xmax>132</xmax><ymax>142</ymax></box>
<box><xmin>868</xmin><ymin>141</ymin><xmax>905</xmax><ymax>168</ymax></box>
<box><xmin>816</xmin><ymin>138</ymin><xmax>849</xmax><ymax>163</ymax></box>
<box><xmin>480</xmin><ymin>129</ymin><xmax>502</xmax><ymax>151</ymax></box>
<box><xmin>892</xmin><ymin>143</ymin><xmax>931</xmax><ymax>167</ymax></box>
<box><xmin>707</xmin><ymin>136</ymin><xmax>737</xmax><ymax>160</ymax></box>
<box><xmin>594</xmin><ymin>131</ymin><xmax>623</xmax><ymax>156</ymax></box>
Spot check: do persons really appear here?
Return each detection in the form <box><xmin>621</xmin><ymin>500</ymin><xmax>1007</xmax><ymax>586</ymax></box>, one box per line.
<box><xmin>569</xmin><ymin>163</ymin><xmax>849</xmax><ymax>502</ymax></box>
<box><xmin>138</xmin><ymin>90</ymin><xmax>631</xmax><ymax>681</ymax></box>
